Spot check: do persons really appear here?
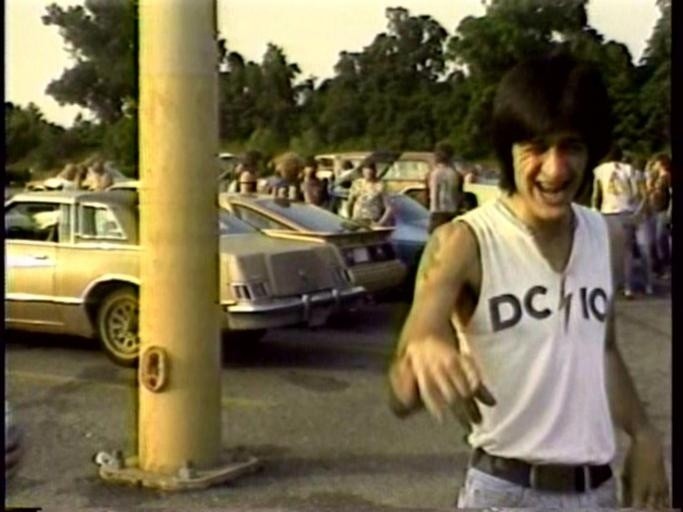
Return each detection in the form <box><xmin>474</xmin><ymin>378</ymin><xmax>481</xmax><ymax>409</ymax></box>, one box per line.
<box><xmin>60</xmin><ymin>157</ymin><xmax>126</xmax><ymax>191</ymax></box>
<box><xmin>386</xmin><ymin>60</ymin><xmax>671</xmax><ymax>511</ymax></box>
<box><xmin>227</xmin><ymin>150</ymin><xmax>393</xmax><ymax>227</ymax></box>
<box><xmin>423</xmin><ymin>142</ymin><xmax>465</xmax><ymax>235</ymax></box>
<box><xmin>586</xmin><ymin>140</ymin><xmax>670</xmax><ymax>300</ymax></box>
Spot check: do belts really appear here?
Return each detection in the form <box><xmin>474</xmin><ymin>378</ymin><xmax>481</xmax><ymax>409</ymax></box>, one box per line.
<box><xmin>471</xmin><ymin>447</ymin><xmax>612</xmax><ymax>495</ymax></box>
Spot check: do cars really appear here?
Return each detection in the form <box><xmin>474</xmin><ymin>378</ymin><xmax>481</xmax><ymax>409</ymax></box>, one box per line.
<box><xmin>300</xmin><ymin>150</ymin><xmax>512</xmax><ymax>278</ymax></box>
<box><xmin>28</xmin><ymin>180</ymin><xmax>409</xmax><ymax>331</ymax></box>
<box><xmin>3</xmin><ymin>190</ymin><xmax>367</xmax><ymax>363</ymax></box>
